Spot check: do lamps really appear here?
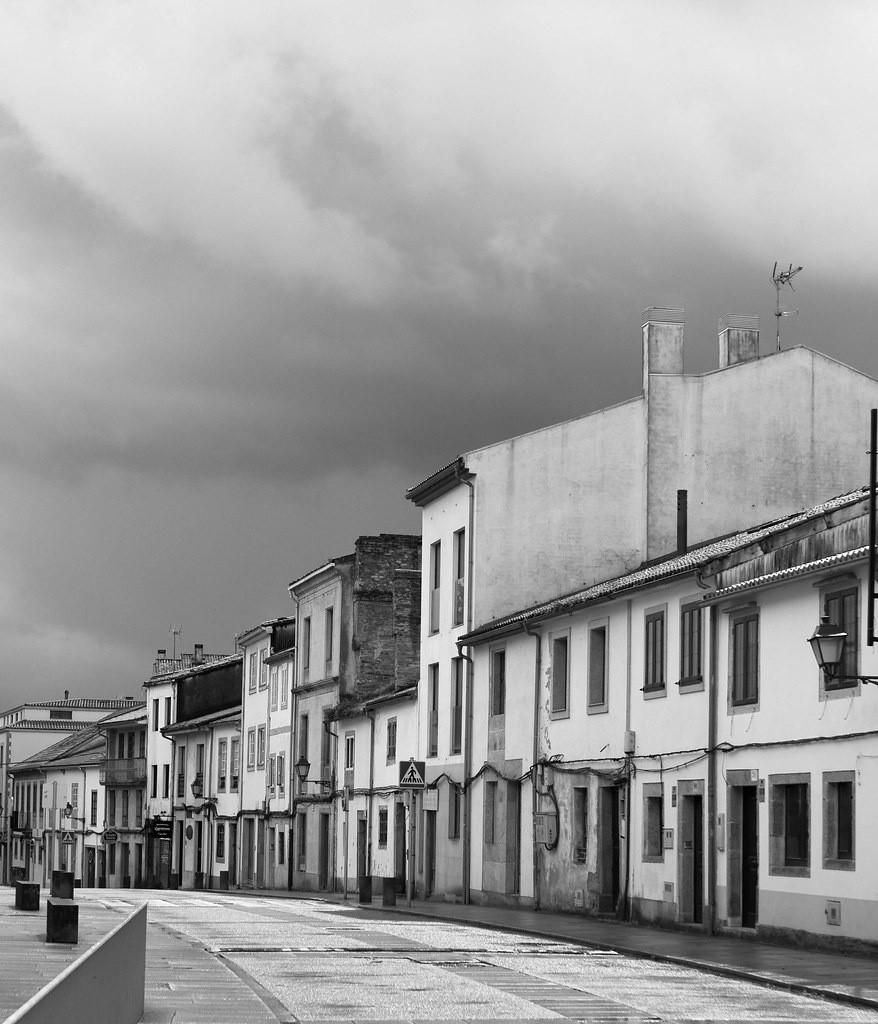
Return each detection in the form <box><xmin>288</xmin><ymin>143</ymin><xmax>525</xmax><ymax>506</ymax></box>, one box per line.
<box><xmin>64</xmin><ymin>802</ymin><xmax>85</xmax><ymax>824</ymax></box>
<box><xmin>295</xmin><ymin>755</ymin><xmax>332</xmax><ymax>789</ymax></box>
<box><xmin>808</xmin><ymin>615</ymin><xmax>878</xmax><ymax>685</ymax></box>
<box><xmin>191</xmin><ymin>779</ymin><xmax>217</xmax><ymax>803</ymax></box>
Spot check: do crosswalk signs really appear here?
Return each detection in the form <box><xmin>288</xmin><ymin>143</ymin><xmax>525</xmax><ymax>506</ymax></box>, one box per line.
<box><xmin>399</xmin><ymin>761</ymin><xmax>427</xmax><ymax>790</ymax></box>
